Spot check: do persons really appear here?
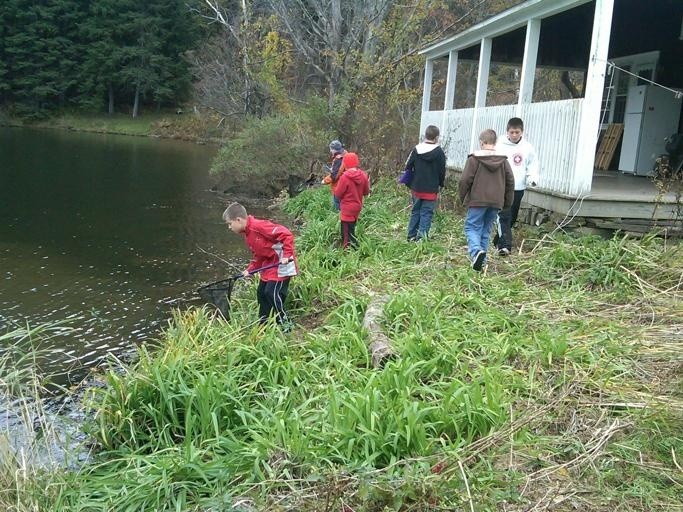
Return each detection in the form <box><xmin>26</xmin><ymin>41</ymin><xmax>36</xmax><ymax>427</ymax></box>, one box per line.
<box><xmin>321</xmin><ymin>140</ymin><xmax>370</xmax><ymax>249</ymax></box>
<box><xmin>403</xmin><ymin>125</ymin><xmax>446</xmax><ymax>244</ymax></box>
<box><xmin>458</xmin><ymin>118</ymin><xmax>540</xmax><ymax>271</ymax></box>
<box><xmin>222</xmin><ymin>202</ymin><xmax>298</xmax><ymax>335</ymax></box>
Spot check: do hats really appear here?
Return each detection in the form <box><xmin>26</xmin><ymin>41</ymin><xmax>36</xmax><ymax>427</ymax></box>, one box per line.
<box><xmin>329</xmin><ymin>140</ymin><xmax>344</xmax><ymax>153</ymax></box>
<box><xmin>343</xmin><ymin>153</ymin><xmax>360</xmax><ymax>168</ymax></box>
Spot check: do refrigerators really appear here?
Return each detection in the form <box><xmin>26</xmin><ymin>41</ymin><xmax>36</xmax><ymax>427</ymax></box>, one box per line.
<box><xmin>618</xmin><ymin>85</ymin><xmax>683</xmax><ymax>177</ymax></box>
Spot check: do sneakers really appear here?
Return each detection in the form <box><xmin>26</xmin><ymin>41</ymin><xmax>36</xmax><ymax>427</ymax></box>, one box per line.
<box><xmin>495</xmin><ymin>243</ymin><xmax>511</xmax><ymax>256</ymax></box>
<box><xmin>472</xmin><ymin>250</ymin><xmax>486</xmax><ymax>271</ymax></box>
